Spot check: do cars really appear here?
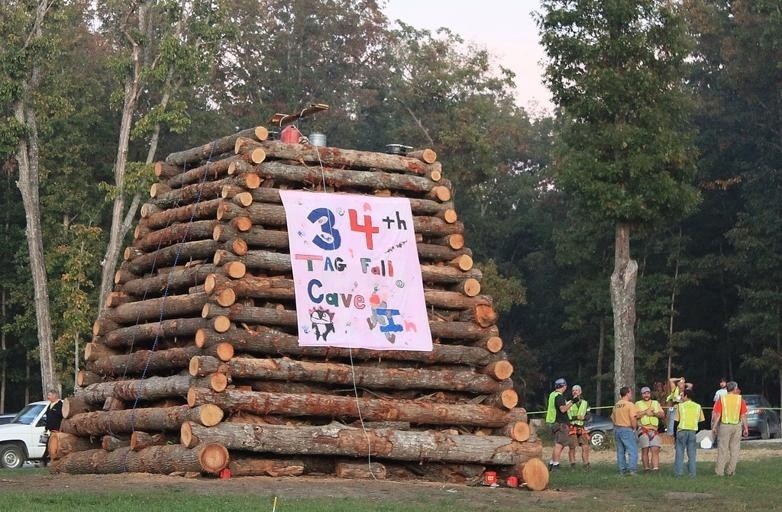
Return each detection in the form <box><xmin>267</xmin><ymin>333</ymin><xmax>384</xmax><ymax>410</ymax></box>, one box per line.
<box><xmin>0</xmin><ymin>412</ymin><xmax>46</xmax><ymax>428</ymax></box>
<box><xmin>582</xmin><ymin>414</ymin><xmax>614</xmax><ymax>452</ymax></box>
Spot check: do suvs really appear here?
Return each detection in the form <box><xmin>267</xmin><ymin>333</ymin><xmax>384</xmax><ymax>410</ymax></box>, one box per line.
<box><xmin>741</xmin><ymin>394</ymin><xmax>780</xmax><ymax>440</ymax></box>
<box><xmin>0</xmin><ymin>399</ymin><xmax>65</xmax><ymax>468</ymax></box>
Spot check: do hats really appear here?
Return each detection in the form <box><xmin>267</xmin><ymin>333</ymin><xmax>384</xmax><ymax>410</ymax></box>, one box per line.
<box><xmin>641</xmin><ymin>387</ymin><xmax>650</xmax><ymax>394</ymax></box>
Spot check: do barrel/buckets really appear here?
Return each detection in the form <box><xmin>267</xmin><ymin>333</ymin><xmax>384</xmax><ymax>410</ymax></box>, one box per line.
<box><xmin>309</xmin><ymin>133</ymin><xmax>327</xmax><ymax>147</ymax></box>
<box><xmin>281</xmin><ymin>125</ymin><xmax>300</xmax><ymax>144</ymax></box>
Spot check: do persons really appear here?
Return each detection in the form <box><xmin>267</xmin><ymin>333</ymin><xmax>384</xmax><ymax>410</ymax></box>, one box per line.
<box><xmin>39</xmin><ymin>389</ymin><xmax>63</xmax><ymax>467</ymax></box>
<box><xmin>545</xmin><ymin>377</ymin><xmax>751</xmax><ymax>477</ymax></box>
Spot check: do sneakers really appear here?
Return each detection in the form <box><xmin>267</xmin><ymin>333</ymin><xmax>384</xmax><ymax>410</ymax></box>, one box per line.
<box><xmin>549</xmin><ymin>462</ymin><xmax>559</xmax><ymax>472</ymax></box>
